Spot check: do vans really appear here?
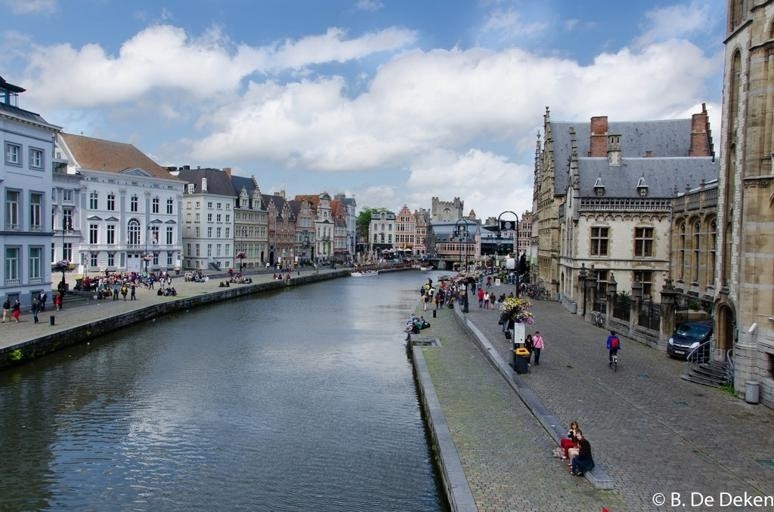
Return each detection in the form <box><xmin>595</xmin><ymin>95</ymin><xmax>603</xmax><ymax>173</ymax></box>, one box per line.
<box><xmin>666</xmin><ymin>320</ymin><xmax>710</xmax><ymax>364</ymax></box>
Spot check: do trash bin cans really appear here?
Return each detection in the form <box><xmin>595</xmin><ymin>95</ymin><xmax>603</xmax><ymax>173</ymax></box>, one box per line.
<box><xmin>745</xmin><ymin>380</ymin><xmax>760</xmax><ymax>403</ymax></box>
<box><xmin>514</xmin><ymin>347</ymin><xmax>530</xmax><ymax>374</ymax></box>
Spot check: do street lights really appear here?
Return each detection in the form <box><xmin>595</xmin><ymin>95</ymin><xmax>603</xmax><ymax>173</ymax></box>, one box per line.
<box><xmin>62</xmin><ymin>223</ymin><xmax>76</xmax><ymax>295</ymax></box>
<box><xmin>239</xmin><ymin>225</ymin><xmax>249</xmax><ymax>272</ymax></box>
<box><xmin>144</xmin><ymin>220</ymin><xmax>158</xmax><ymax>276</ymax></box>
<box><xmin>450</xmin><ymin>218</ymin><xmax>471</xmax><ymax>277</ymax></box>
<box><xmin>495</xmin><ymin>210</ymin><xmax>520</xmax><ymax>305</ymax></box>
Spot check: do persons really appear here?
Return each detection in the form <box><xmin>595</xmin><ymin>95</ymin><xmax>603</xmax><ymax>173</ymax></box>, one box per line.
<box><xmin>273</xmin><ymin>272</ymin><xmax>291</xmax><ymax>279</ymax></box>
<box><xmin>83</xmin><ymin>272</ymin><xmax>176</xmax><ymax>301</ymax></box>
<box><xmin>607</xmin><ymin>330</ymin><xmax>621</xmax><ymax>365</ymax></box>
<box><xmin>561</xmin><ymin>422</ymin><xmax>594</xmax><ymax>476</ymax></box>
<box><xmin>406</xmin><ymin>313</ymin><xmax>430</xmax><ymax>341</ymax></box>
<box><xmin>184</xmin><ymin>268</ymin><xmax>210</xmax><ymax>282</ymax></box>
<box><xmin>30</xmin><ymin>290</ymin><xmax>47</xmax><ymax>324</ymax></box>
<box><xmin>524</xmin><ymin>331</ymin><xmax>544</xmax><ymax>367</ymax></box>
<box><xmin>1</xmin><ymin>298</ymin><xmax>21</xmax><ymax>323</ymax></box>
<box><xmin>420</xmin><ymin>264</ymin><xmax>525</xmax><ymax>312</ymax></box>
<box><xmin>55</xmin><ymin>286</ymin><xmax>65</xmax><ymax>312</ymax></box>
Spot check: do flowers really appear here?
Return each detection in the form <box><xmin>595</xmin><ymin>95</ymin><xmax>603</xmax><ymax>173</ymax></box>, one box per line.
<box><xmin>56</xmin><ymin>261</ymin><xmax>75</xmax><ymax>272</ymax></box>
<box><xmin>499</xmin><ymin>296</ymin><xmax>534</xmax><ymax>324</ymax></box>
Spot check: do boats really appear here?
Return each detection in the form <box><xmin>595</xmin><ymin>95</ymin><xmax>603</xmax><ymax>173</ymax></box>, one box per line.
<box><xmin>418</xmin><ymin>264</ymin><xmax>434</xmax><ymax>271</ymax></box>
<box><xmin>351</xmin><ymin>269</ymin><xmax>380</xmax><ymax>277</ymax></box>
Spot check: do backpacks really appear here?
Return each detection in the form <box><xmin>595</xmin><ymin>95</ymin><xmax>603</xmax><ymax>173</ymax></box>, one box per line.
<box><xmin>612</xmin><ymin>338</ymin><xmax>619</xmax><ymax>347</ymax></box>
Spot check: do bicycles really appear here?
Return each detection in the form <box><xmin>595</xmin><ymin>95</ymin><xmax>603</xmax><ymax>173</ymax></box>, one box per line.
<box><xmin>605</xmin><ymin>346</ymin><xmax>620</xmax><ymax>370</ymax></box>
<box><xmin>591</xmin><ymin>311</ymin><xmax>604</xmax><ymax>328</ymax></box>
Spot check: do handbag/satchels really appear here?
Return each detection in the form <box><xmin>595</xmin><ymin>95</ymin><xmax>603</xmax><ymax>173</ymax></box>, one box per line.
<box><xmin>532</xmin><ymin>346</ymin><xmax>535</xmax><ymax>351</ymax></box>
